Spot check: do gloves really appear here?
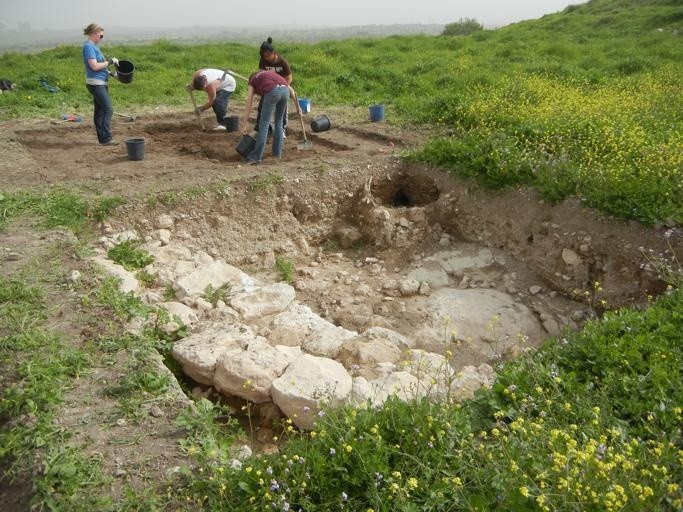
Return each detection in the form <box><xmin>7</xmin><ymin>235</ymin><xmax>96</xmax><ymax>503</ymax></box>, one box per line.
<box><xmin>107</xmin><ymin>57</ymin><xmax>120</xmax><ymax>67</ymax></box>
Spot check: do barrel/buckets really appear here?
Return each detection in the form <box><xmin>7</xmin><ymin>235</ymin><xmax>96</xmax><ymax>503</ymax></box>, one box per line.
<box><xmin>309</xmin><ymin>114</ymin><xmax>331</xmax><ymax>132</ymax></box>
<box><xmin>298</xmin><ymin>99</ymin><xmax>313</xmax><ymax>112</ymax></box>
<box><xmin>222</xmin><ymin>116</ymin><xmax>241</xmax><ymax>132</ymax></box>
<box><xmin>234</xmin><ymin>134</ymin><xmax>257</xmax><ymax>156</ymax></box>
<box><xmin>368</xmin><ymin>105</ymin><xmax>386</xmax><ymax>121</ymax></box>
<box><xmin>116</xmin><ymin>60</ymin><xmax>135</xmax><ymax>84</ymax></box>
<box><xmin>125</xmin><ymin>138</ymin><xmax>145</xmax><ymax>160</ymax></box>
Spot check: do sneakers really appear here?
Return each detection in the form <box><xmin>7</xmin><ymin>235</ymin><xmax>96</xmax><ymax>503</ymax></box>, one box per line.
<box><xmin>212</xmin><ymin>124</ymin><xmax>227</xmax><ymax>131</ymax></box>
<box><xmin>99</xmin><ymin>138</ymin><xmax>120</xmax><ymax>146</ymax></box>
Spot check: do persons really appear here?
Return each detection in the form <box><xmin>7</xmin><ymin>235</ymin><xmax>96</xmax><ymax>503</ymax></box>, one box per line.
<box><xmin>186</xmin><ymin>69</ymin><xmax>235</xmax><ymax>130</ymax></box>
<box><xmin>81</xmin><ymin>23</ymin><xmax>120</xmax><ymax>145</ymax></box>
<box><xmin>241</xmin><ymin>70</ymin><xmax>300</xmax><ymax>165</ymax></box>
<box><xmin>247</xmin><ymin>37</ymin><xmax>292</xmax><ymax>139</ymax></box>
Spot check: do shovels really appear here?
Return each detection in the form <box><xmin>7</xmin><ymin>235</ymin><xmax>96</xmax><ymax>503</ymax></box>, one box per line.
<box><xmin>295</xmin><ymin>91</ymin><xmax>312</xmax><ymax>151</ymax></box>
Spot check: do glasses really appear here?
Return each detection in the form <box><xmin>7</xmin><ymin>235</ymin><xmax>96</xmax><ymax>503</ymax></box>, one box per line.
<box><xmin>96</xmin><ymin>34</ymin><xmax>103</xmax><ymax>40</ymax></box>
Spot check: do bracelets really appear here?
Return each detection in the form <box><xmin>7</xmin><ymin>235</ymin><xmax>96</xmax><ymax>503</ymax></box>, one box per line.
<box><xmin>107</xmin><ymin>59</ymin><xmax>113</xmax><ymax>65</ymax></box>
